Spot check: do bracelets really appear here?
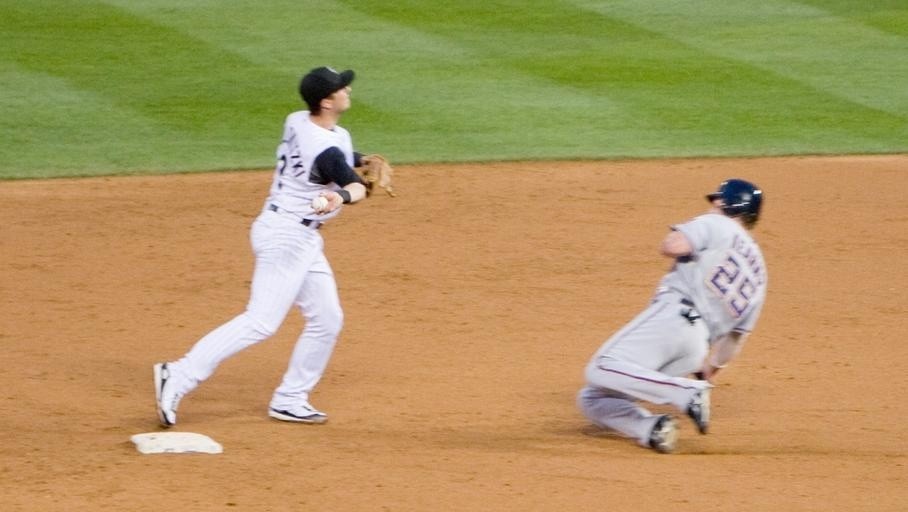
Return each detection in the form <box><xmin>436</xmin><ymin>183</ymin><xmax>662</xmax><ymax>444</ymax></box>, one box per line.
<box><xmin>332</xmin><ymin>188</ymin><xmax>353</xmax><ymax>206</ymax></box>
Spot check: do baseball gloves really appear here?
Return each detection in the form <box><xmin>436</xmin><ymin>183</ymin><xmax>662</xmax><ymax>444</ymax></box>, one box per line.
<box><xmin>360</xmin><ymin>153</ymin><xmax>395</xmax><ymax>199</ymax></box>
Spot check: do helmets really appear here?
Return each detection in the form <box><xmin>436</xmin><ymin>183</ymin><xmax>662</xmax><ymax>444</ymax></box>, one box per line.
<box><xmin>708</xmin><ymin>180</ymin><xmax>762</xmax><ymax>222</ymax></box>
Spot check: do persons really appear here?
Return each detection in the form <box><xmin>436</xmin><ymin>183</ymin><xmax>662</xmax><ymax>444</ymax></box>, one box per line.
<box><xmin>152</xmin><ymin>66</ymin><xmax>397</xmax><ymax>430</ymax></box>
<box><xmin>577</xmin><ymin>178</ymin><xmax>772</xmax><ymax>454</ymax></box>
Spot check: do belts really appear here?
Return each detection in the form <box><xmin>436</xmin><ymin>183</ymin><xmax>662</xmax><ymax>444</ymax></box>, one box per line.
<box><xmin>682</xmin><ymin>298</ymin><xmax>694</xmax><ymax>306</ymax></box>
<box><xmin>269</xmin><ymin>204</ymin><xmax>323</xmax><ymax>229</ymax></box>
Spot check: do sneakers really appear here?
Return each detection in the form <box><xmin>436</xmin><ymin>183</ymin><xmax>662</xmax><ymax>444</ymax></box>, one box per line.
<box><xmin>154</xmin><ymin>361</ymin><xmax>181</xmax><ymax>425</ymax></box>
<box><xmin>651</xmin><ymin>415</ymin><xmax>677</xmax><ymax>451</ymax></box>
<box><xmin>268</xmin><ymin>403</ymin><xmax>328</xmax><ymax>424</ymax></box>
<box><xmin>689</xmin><ymin>381</ymin><xmax>710</xmax><ymax>434</ymax></box>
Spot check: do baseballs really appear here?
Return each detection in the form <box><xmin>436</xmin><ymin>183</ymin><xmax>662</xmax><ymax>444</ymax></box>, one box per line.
<box><xmin>311</xmin><ymin>196</ymin><xmax>329</xmax><ymax>212</ymax></box>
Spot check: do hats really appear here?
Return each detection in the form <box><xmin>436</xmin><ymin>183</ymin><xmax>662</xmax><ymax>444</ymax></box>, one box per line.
<box><xmin>301</xmin><ymin>67</ymin><xmax>354</xmax><ymax>104</ymax></box>
